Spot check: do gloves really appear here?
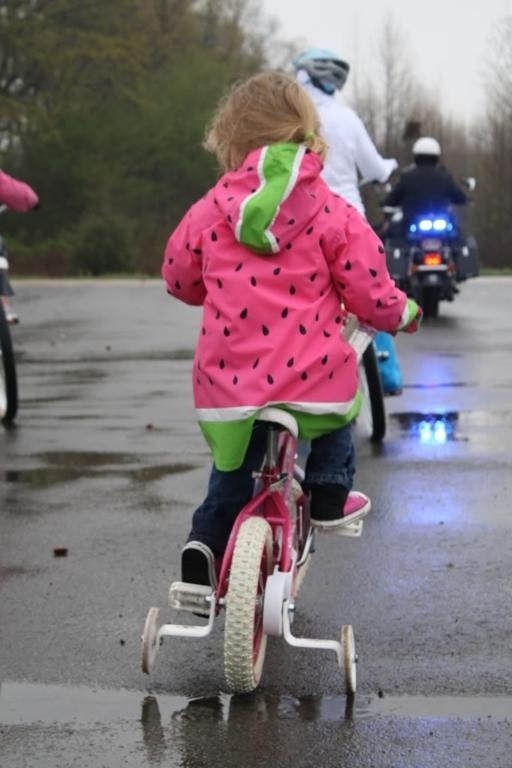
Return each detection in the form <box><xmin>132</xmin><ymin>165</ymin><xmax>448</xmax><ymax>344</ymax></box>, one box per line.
<box><xmin>392</xmin><ymin>299</ymin><xmax>427</xmax><ymax>337</ymax></box>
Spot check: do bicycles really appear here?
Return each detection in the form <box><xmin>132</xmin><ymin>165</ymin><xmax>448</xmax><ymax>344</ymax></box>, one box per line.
<box><xmin>1</xmin><ymin>194</ymin><xmax>42</xmax><ymax>431</ymax></box>
<box><xmin>128</xmin><ymin>252</ymin><xmax>383</xmax><ymax>701</ymax></box>
<box><xmin>320</xmin><ymin>160</ymin><xmax>398</xmax><ymax>452</ymax></box>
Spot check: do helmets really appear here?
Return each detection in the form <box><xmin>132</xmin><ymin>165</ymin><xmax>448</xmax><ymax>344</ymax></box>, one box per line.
<box><xmin>410</xmin><ymin>137</ymin><xmax>443</xmax><ymax>156</ymax></box>
<box><xmin>291</xmin><ymin>48</ymin><xmax>349</xmax><ymax>95</ymax></box>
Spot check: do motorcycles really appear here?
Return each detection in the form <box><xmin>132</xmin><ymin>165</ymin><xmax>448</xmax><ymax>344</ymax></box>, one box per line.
<box><xmin>380</xmin><ymin>176</ymin><xmax>484</xmax><ymax>325</ymax></box>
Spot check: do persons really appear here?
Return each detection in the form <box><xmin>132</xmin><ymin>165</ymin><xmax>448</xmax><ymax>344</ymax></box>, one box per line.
<box><xmin>378</xmin><ymin>136</ymin><xmax>471</xmax><ymax>244</ymax></box>
<box><xmin>162</xmin><ymin>73</ymin><xmax>425</xmax><ymax>620</ymax></box>
<box><xmin>290</xmin><ymin>46</ymin><xmax>404</xmax><ymax>397</ymax></box>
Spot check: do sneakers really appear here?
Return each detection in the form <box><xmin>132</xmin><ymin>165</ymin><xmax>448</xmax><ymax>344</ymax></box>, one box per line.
<box><xmin>310</xmin><ymin>491</ymin><xmax>372</xmax><ymax>529</ymax></box>
<box><xmin>178</xmin><ymin>539</ymin><xmax>223</xmax><ymax>621</ymax></box>
